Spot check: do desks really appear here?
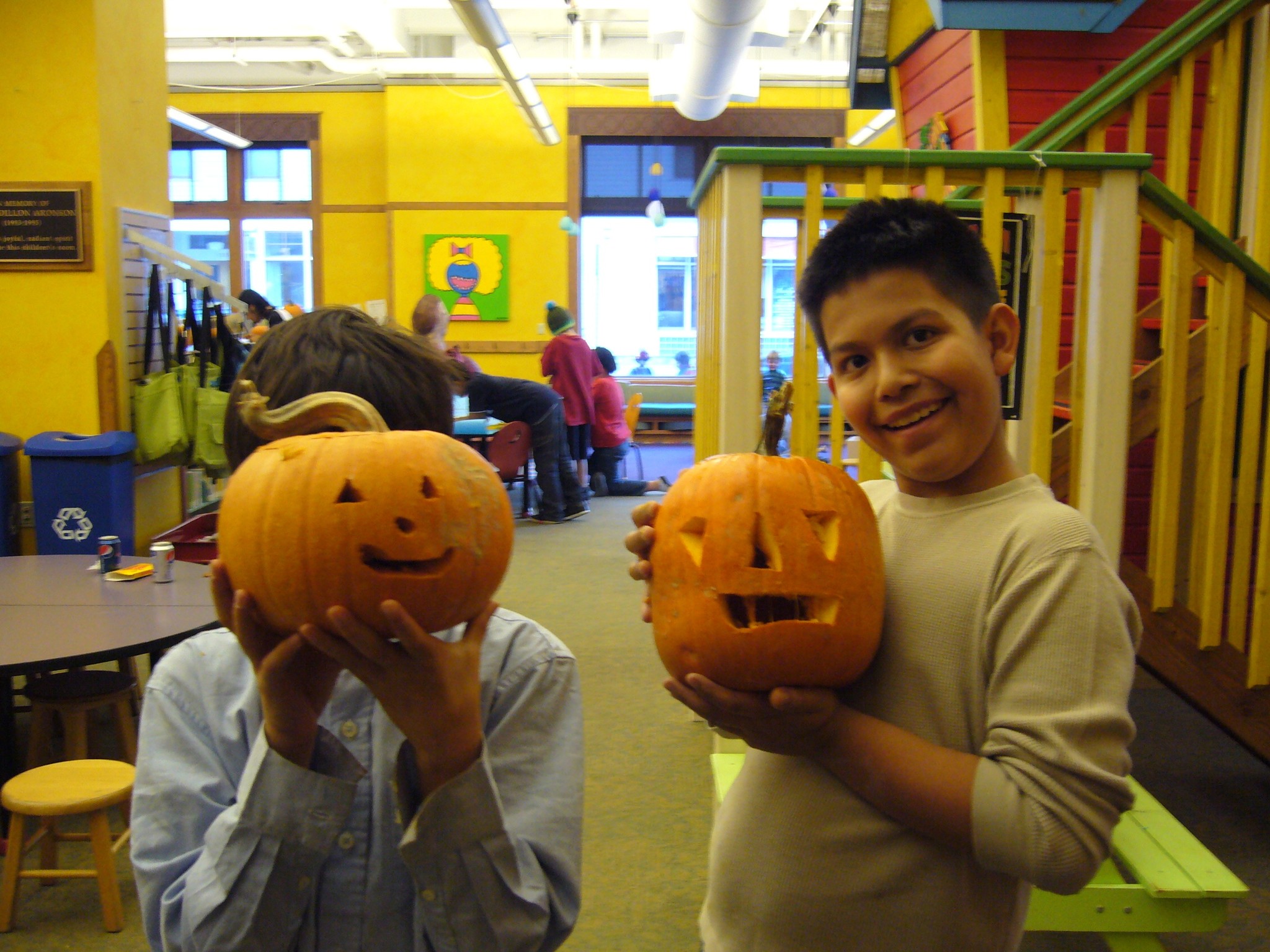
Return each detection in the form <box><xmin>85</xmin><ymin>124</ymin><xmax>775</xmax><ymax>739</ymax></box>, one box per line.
<box><xmin>712</xmin><ymin>753</ymin><xmax>1248</xmax><ymax>951</ymax></box>
<box><xmin>0</xmin><ymin>552</ymin><xmax>222</xmax><ymax>841</ymax></box>
<box><xmin>453</xmin><ymin>417</ymin><xmax>507</xmax><ymax>460</ymax></box>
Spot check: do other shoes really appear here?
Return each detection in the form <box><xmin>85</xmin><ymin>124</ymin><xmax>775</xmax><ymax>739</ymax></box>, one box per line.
<box><xmin>658</xmin><ymin>476</ymin><xmax>671</xmax><ymax>493</ymax></box>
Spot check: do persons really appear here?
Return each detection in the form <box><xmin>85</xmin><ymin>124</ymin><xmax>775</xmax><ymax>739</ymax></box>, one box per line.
<box><xmin>128</xmin><ymin>306</ymin><xmax>584</xmax><ymax>952</ymax></box>
<box><xmin>621</xmin><ymin>200</ymin><xmax>1145</xmax><ymax>952</ymax></box>
<box><xmin>237</xmin><ymin>288</ymin><xmax>288</xmax><ymax>345</ymax></box>
<box><xmin>440</xmin><ymin>356</ymin><xmax>591</xmax><ymax>526</ymax></box>
<box><xmin>585</xmin><ymin>347</ymin><xmax>671</xmax><ymax>496</ymax></box>
<box><xmin>760</xmin><ymin>351</ymin><xmax>792</xmax><ymax>435</ymax></box>
<box><xmin>631</xmin><ymin>352</ymin><xmax>653</xmax><ymax>378</ymax></box>
<box><xmin>674</xmin><ymin>352</ymin><xmax>696</xmax><ymax>376</ymax></box>
<box><xmin>542</xmin><ymin>302</ymin><xmax>603</xmax><ymax>505</ymax></box>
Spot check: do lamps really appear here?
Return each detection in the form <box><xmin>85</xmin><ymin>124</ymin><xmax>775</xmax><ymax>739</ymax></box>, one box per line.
<box><xmin>449</xmin><ymin>1</ymin><xmax>560</xmax><ymax>148</ymax></box>
<box><xmin>167</xmin><ymin>1</ymin><xmax>253</xmax><ymax>149</ymax></box>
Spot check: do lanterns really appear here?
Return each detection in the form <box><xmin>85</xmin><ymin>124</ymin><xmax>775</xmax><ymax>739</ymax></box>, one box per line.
<box><xmin>646</xmin><ymin>383</ymin><xmax>889</xmax><ymax>697</ymax></box>
<box><xmin>216</xmin><ymin>374</ymin><xmax>513</xmax><ymax>641</ymax></box>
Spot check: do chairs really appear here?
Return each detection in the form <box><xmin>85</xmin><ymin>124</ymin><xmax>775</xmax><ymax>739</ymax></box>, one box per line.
<box><xmin>483</xmin><ymin>420</ymin><xmax>533</xmax><ymax>518</ymax></box>
<box><xmin>623</xmin><ymin>392</ymin><xmax>645</xmax><ymax>482</ymax></box>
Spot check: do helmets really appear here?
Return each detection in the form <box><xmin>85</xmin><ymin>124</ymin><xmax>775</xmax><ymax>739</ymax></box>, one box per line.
<box><xmin>635</xmin><ymin>350</ymin><xmax>649</xmax><ymax>359</ymax></box>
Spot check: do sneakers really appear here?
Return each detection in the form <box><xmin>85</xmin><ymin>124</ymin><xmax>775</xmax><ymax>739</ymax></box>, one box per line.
<box><xmin>531</xmin><ymin>511</ymin><xmax>565</xmax><ymax>524</ymax></box>
<box><xmin>560</xmin><ymin>501</ymin><xmax>591</xmax><ymax>520</ymax></box>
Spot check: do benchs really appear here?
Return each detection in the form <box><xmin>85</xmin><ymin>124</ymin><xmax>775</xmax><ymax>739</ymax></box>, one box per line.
<box><xmin>621</xmin><ymin>402</ymin><xmax>857</xmax><ymax>445</ymax></box>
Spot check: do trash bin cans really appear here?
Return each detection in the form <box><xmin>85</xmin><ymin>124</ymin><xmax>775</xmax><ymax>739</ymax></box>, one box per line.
<box><xmin>21</xmin><ymin>429</ymin><xmax>137</xmax><ymax>561</ymax></box>
<box><xmin>0</xmin><ymin>432</ymin><xmax>25</xmax><ymax>560</ymax></box>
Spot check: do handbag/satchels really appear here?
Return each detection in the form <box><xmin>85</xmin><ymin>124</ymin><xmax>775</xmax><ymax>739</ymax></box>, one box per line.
<box><xmin>190</xmin><ymin>285</ymin><xmax>238</xmax><ymax>476</ymax></box>
<box><xmin>176</xmin><ymin>279</ymin><xmax>221</xmax><ymax>445</ymax></box>
<box><xmin>164</xmin><ymin>285</ymin><xmax>196</xmax><ymax>443</ymax></box>
<box><xmin>132</xmin><ymin>264</ymin><xmax>190</xmax><ymax>465</ymax></box>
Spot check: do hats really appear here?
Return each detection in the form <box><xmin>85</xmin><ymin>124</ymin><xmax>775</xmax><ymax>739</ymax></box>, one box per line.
<box><xmin>546</xmin><ymin>301</ymin><xmax>576</xmax><ymax>335</ymax></box>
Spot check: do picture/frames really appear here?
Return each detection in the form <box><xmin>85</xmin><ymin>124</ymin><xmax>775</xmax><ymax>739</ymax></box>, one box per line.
<box><xmin>0</xmin><ymin>182</ymin><xmax>96</xmax><ymax>272</ymax></box>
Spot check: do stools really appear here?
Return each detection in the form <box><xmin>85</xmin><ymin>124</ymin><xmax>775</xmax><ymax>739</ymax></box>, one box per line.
<box><xmin>0</xmin><ymin>758</ymin><xmax>138</xmax><ymax>933</ymax></box>
<box><xmin>24</xmin><ymin>671</ymin><xmax>138</xmax><ymax>830</ymax></box>
